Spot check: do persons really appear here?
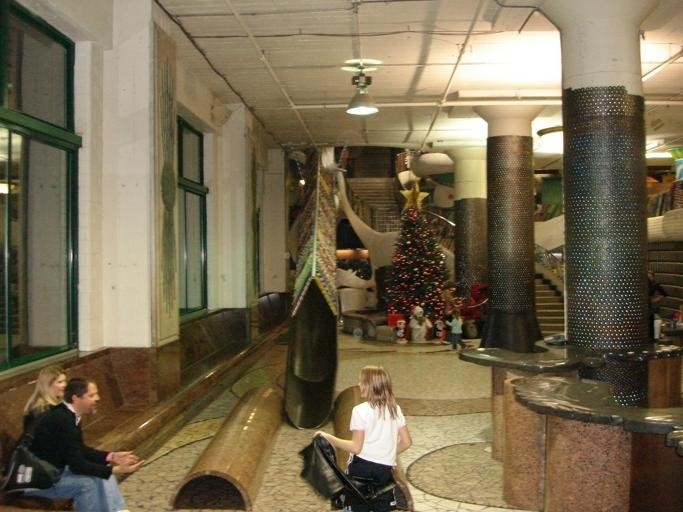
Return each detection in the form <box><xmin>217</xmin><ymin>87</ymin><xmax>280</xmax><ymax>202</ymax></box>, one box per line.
<box><xmin>445</xmin><ymin>309</ymin><xmax>467</xmax><ymax>351</ymax></box>
<box><xmin>21</xmin><ymin>365</ymin><xmax>127</xmax><ymax>512</ymax></box>
<box><xmin>6</xmin><ymin>376</ymin><xmax>146</xmax><ymax>512</ymax></box>
<box><xmin>648</xmin><ymin>271</ymin><xmax>667</xmax><ymax>315</ymax></box>
<box><xmin>312</xmin><ymin>365</ymin><xmax>413</xmax><ymax>511</ymax></box>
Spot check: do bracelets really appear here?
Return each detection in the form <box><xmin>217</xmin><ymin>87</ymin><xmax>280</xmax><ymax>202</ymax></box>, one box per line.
<box><xmin>109</xmin><ymin>451</ymin><xmax>114</xmax><ymax>462</ymax></box>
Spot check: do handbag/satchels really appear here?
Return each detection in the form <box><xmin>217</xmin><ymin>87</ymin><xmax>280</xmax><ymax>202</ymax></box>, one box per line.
<box><xmin>1</xmin><ymin>441</ymin><xmax>63</xmax><ymax>494</ymax></box>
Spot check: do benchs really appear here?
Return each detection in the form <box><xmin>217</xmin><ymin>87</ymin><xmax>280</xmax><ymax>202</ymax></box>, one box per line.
<box><xmin>0</xmin><ymin>292</ymin><xmax>292</xmax><ymax>512</ymax></box>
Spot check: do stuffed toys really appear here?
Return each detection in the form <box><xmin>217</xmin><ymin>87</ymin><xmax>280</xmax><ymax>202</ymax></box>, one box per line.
<box><xmin>433</xmin><ymin>318</ymin><xmax>445</xmax><ymax>339</ymax></box>
<box><xmin>408</xmin><ymin>305</ymin><xmax>434</xmax><ymax>341</ymax></box>
<box><xmin>393</xmin><ymin>317</ymin><xmax>407</xmax><ymax>342</ymax></box>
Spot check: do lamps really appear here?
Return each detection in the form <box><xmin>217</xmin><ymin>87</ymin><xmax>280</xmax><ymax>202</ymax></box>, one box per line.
<box><xmin>346</xmin><ymin>66</ymin><xmax>379</xmax><ymax>115</ymax></box>
